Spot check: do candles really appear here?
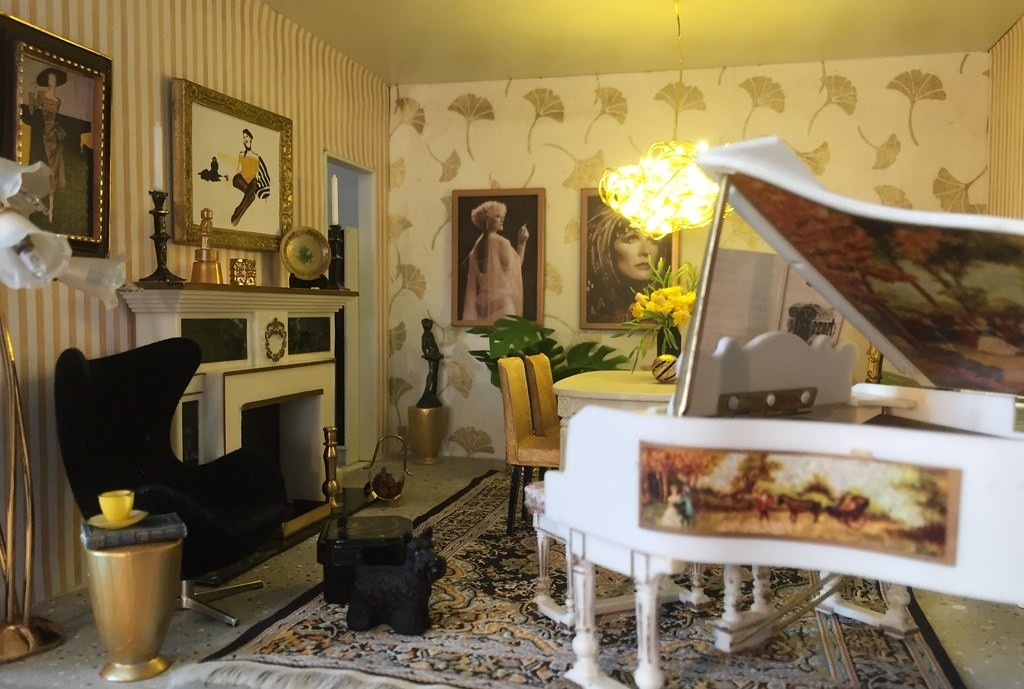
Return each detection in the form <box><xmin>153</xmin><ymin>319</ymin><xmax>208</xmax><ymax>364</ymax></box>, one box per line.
<box><xmin>153</xmin><ymin>124</ymin><xmax>163</xmax><ymax>192</ymax></box>
<box><xmin>331</xmin><ymin>174</ymin><xmax>339</xmax><ymax>225</ymax></box>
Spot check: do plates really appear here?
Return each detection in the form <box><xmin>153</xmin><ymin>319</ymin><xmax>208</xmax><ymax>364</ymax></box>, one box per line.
<box><xmin>86</xmin><ymin>510</ymin><xmax>149</xmax><ymax>529</ymax></box>
<box><xmin>269</xmin><ymin>226</ymin><xmax>331</xmax><ymax>280</ymax></box>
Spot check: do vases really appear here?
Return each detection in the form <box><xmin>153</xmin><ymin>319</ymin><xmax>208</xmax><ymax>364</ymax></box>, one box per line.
<box><xmin>579</xmin><ymin>188</ymin><xmax>681</xmax><ymax>331</ymax></box>
<box><xmin>657</xmin><ymin>326</ymin><xmax>681</xmax><ymax>358</ymax></box>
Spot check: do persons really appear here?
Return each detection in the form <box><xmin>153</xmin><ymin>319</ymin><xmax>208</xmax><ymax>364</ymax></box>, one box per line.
<box><xmin>463</xmin><ymin>200</ymin><xmax>531</xmax><ymax>325</ymax></box>
<box><xmin>583</xmin><ymin>203</ymin><xmax>671</xmax><ymax>323</ymax></box>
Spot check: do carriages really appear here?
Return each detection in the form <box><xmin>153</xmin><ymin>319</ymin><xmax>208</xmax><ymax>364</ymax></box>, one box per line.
<box><xmin>777</xmin><ymin>490</ymin><xmax>870</xmax><ymax>530</ymax></box>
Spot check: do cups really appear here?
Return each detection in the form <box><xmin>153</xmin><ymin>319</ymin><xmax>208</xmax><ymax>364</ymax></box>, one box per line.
<box><xmin>98</xmin><ymin>490</ymin><xmax>135</xmax><ymax>523</ymax></box>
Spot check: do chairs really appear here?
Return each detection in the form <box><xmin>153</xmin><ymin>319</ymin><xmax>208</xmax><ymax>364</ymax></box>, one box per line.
<box><xmin>498</xmin><ymin>352</ymin><xmax>560</xmax><ymax>537</ymax></box>
<box><xmin>53</xmin><ymin>337</ymin><xmax>287</xmax><ymax>629</ymax></box>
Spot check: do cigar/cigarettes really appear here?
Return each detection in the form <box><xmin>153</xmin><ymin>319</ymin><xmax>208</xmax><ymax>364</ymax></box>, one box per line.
<box><xmin>524</xmin><ymin>222</ymin><xmax>527</xmax><ymax>227</ymax></box>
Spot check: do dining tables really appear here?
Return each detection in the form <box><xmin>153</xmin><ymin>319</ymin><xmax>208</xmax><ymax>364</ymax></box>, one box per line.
<box><xmin>551</xmin><ymin>367</ymin><xmax>675</xmax><ymax>473</ymax></box>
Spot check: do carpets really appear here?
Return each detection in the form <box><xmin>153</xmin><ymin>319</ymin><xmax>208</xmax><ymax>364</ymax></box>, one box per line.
<box><xmin>166</xmin><ymin>470</ymin><xmax>967</xmax><ymax>689</ymax></box>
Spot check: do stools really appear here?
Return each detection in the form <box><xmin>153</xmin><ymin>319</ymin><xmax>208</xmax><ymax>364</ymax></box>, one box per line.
<box><xmin>525</xmin><ymin>481</ymin><xmax>711</xmax><ymax>636</ymax></box>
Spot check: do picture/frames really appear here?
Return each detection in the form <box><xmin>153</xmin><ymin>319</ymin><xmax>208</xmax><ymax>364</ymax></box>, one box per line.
<box><xmin>171</xmin><ymin>75</ymin><xmax>294</xmax><ymax>251</ymax></box>
<box><xmin>451</xmin><ymin>187</ymin><xmax>547</xmax><ymax>328</ymax></box>
<box><xmin>0</xmin><ymin>12</ymin><xmax>114</xmax><ymax>261</ymax></box>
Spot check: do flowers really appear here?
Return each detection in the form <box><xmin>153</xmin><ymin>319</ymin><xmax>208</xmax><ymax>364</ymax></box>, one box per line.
<box><xmin>609</xmin><ymin>253</ymin><xmax>700</xmax><ymax>374</ymax></box>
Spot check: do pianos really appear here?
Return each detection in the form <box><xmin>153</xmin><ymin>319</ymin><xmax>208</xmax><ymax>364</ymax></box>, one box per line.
<box><xmin>534</xmin><ymin>131</ymin><xmax>1024</xmax><ymax>689</ymax></box>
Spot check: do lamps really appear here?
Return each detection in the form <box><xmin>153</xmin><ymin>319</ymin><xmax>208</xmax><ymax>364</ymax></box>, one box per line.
<box><xmin>0</xmin><ymin>155</ymin><xmax>128</xmax><ymax>663</ymax></box>
<box><xmin>598</xmin><ymin>3</ymin><xmax>734</xmax><ymax>239</ymax></box>
